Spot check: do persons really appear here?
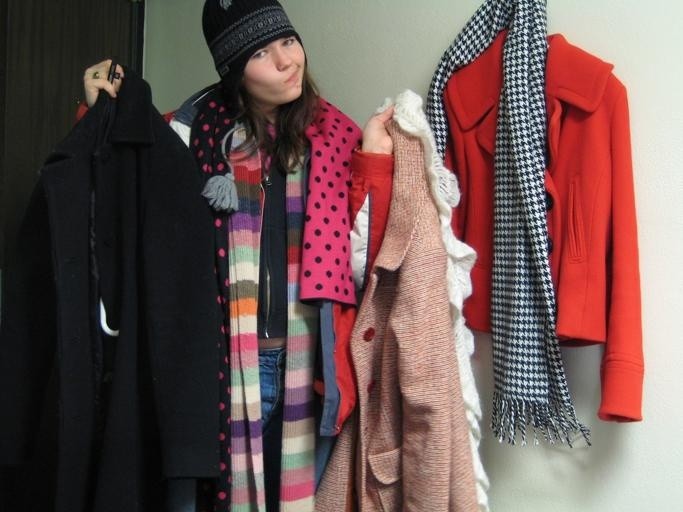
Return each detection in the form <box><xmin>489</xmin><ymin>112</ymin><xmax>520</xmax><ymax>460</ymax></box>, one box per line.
<box><xmin>73</xmin><ymin>1</ymin><xmax>395</xmax><ymax>511</ymax></box>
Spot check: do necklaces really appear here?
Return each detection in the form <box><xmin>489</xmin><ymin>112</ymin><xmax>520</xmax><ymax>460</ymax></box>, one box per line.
<box><xmin>248</xmin><ymin>109</ymin><xmax>283</xmax><ymax>188</ymax></box>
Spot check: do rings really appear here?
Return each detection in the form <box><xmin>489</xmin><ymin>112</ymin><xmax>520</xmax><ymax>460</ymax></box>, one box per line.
<box><xmin>93</xmin><ymin>72</ymin><xmax>99</xmax><ymax>79</ymax></box>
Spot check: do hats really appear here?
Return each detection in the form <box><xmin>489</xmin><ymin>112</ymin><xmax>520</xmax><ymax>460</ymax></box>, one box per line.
<box><xmin>203</xmin><ymin>0</ymin><xmax>299</xmax><ymax>79</ymax></box>
